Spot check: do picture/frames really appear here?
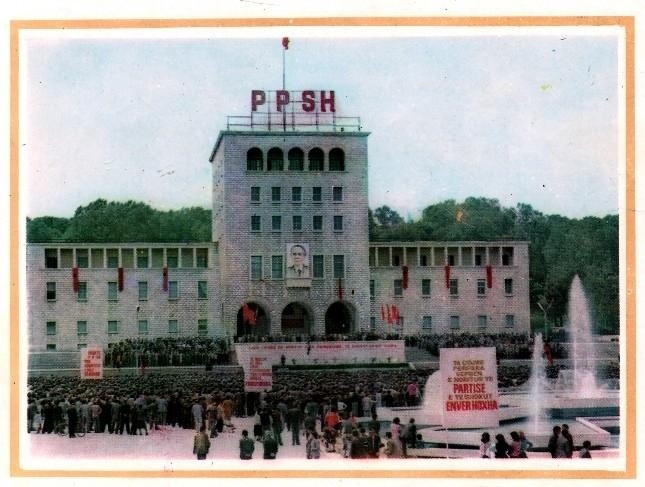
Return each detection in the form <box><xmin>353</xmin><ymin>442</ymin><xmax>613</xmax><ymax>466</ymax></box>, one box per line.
<box><xmin>283</xmin><ymin>241</ymin><xmax>314</xmax><ymax>279</ymax></box>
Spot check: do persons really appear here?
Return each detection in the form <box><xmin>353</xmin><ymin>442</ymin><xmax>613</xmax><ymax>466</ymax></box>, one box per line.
<box><xmin>190</xmin><ymin>369</ymin><xmax>426</xmax><ymax>461</ymax></box>
<box><xmin>285</xmin><ymin>245</ymin><xmax>309</xmax><ymax>278</ymax></box>
<box><xmin>28</xmin><ymin>372</ymin><xmax>254</xmax><ymax>437</ymax></box>
<box><xmin>479</xmin><ymin>424</ymin><xmax>532</xmax><ymax>460</ymax></box>
<box><xmin>395</xmin><ymin>330</ymin><xmax>571</xmax><ymax>391</ymax></box>
<box><xmin>234</xmin><ymin>331</ymin><xmax>401</xmax><ymax>343</ymax></box>
<box><xmin>103</xmin><ymin>332</ymin><xmax>239</xmax><ymax>371</ymax></box>
<box><xmin>548</xmin><ymin>425</ymin><xmax>593</xmax><ymax>459</ymax></box>
